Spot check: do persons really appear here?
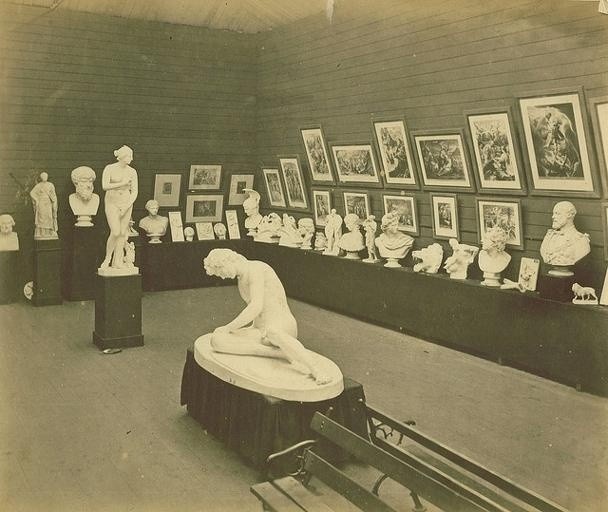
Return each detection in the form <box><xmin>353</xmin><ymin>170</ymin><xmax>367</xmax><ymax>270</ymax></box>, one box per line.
<box><xmin>200</xmin><ymin>244</ymin><xmax>332</xmax><ymax>389</ymax></box>
<box><xmin>29</xmin><ymin>172</ymin><xmax>60</xmax><ymax>240</ymax></box>
<box><xmin>0</xmin><ymin>212</ymin><xmax>21</xmax><ymax>253</ymax></box>
<box><xmin>95</xmin><ymin>146</ymin><xmax>141</xmax><ymax>277</ymax></box>
<box><xmin>68</xmin><ymin>165</ymin><xmax>100</xmax><ymax>218</ymax></box>
<box><xmin>138</xmin><ymin>189</ymin><xmax>594</xmax><ymax>292</ymax></box>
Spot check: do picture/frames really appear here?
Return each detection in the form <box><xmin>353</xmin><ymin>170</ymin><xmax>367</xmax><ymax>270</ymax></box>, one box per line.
<box><xmin>153</xmin><ymin>173</ymin><xmax>182</xmax><ymax>207</ymax></box>
<box><xmin>183</xmin><ymin>192</ymin><xmax>226</xmax><ymax>224</ymax></box>
<box><xmin>226</xmin><ymin>172</ymin><xmax>256</xmax><ymax>208</ymax></box>
<box><xmin>187</xmin><ymin>162</ymin><xmax>224</xmax><ymax>191</ymax></box>
